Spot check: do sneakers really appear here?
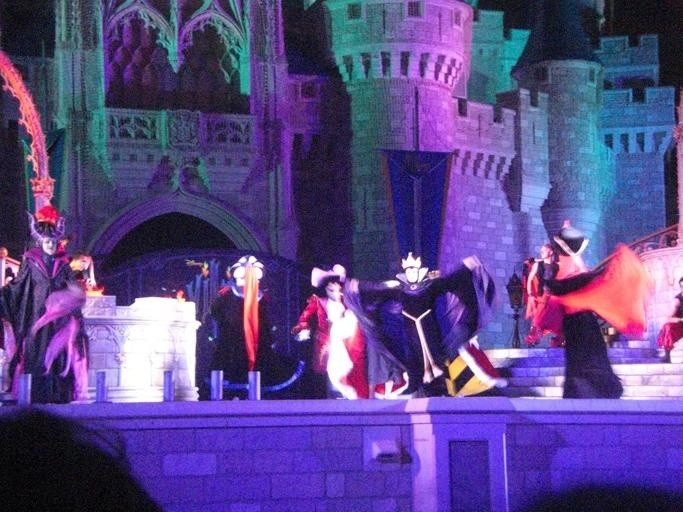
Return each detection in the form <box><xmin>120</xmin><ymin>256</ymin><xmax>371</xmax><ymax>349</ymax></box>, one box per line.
<box><xmin>651</xmin><ymin>352</ymin><xmax>670</xmax><ymax>364</ymax></box>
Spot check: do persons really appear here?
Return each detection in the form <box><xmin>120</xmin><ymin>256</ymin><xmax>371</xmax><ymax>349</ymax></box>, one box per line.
<box><xmin>522</xmin><ymin>242</ymin><xmax>566</xmax><ymax>349</ymax></box>
<box><xmin>345</xmin><ymin>251</ymin><xmax>508</xmax><ymax>398</ymax></box>
<box><xmin>654</xmin><ymin>276</ymin><xmax>683</xmax><ymax>364</ymax></box>
<box><xmin>522</xmin><ymin>254</ymin><xmax>623</xmax><ymax>400</ymax></box>
<box><xmin>68</xmin><ymin>250</ymin><xmax>95</xmax><ymax>289</ymax></box>
<box><xmin>1</xmin><ymin>236</ymin><xmax>89</xmax><ymax>404</ymax></box>
<box><xmin>288</xmin><ymin>264</ymin><xmax>363</xmax><ymax>399</ymax></box>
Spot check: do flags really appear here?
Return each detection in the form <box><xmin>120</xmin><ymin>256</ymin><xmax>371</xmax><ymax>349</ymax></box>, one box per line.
<box><xmin>240</xmin><ymin>261</ymin><xmax>260</xmax><ymax>372</ymax></box>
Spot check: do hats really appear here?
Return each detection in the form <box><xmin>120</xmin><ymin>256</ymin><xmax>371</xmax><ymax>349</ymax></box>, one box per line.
<box><xmin>553</xmin><ymin>221</ymin><xmax>589</xmax><ymax>256</ymax></box>
<box><xmin>311</xmin><ymin>264</ymin><xmax>346</xmax><ymax>288</ymax></box>
<box><xmin>26</xmin><ymin>204</ymin><xmax>64</xmax><ymax>238</ymax></box>
<box><xmin>230</xmin><ymin>254</ymin><xmax>268</xmax><ymax>277</ymax></box>
